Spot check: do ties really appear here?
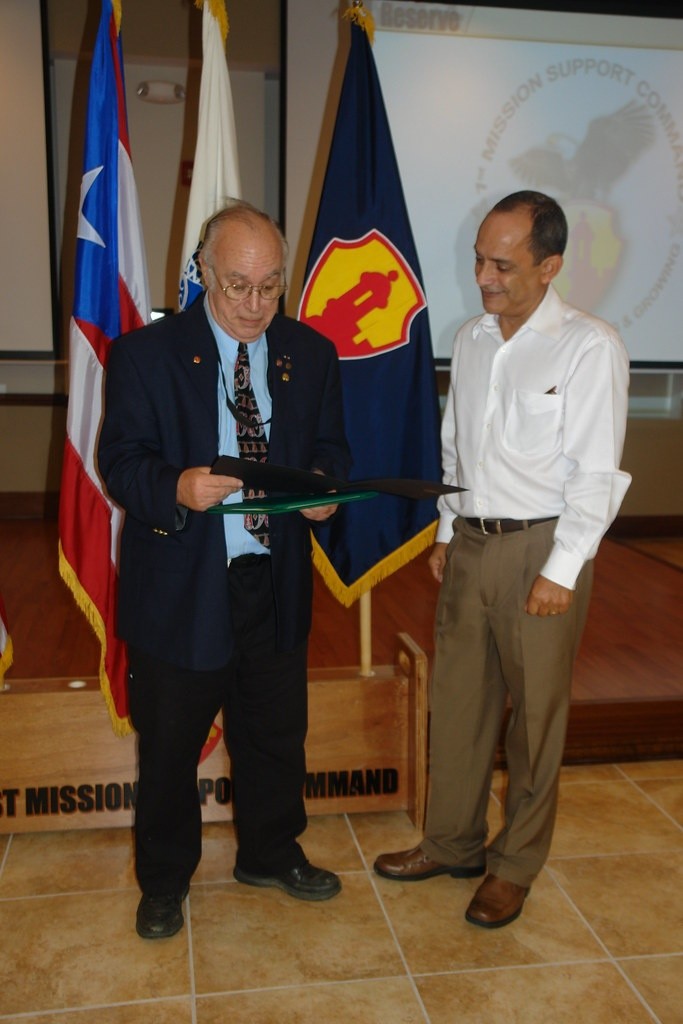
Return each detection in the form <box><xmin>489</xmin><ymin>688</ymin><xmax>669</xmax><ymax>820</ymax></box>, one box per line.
<box><xmin>235</xmin><ymin>343</ymin><xmax>270</xmax><ymax>549</ymax></box>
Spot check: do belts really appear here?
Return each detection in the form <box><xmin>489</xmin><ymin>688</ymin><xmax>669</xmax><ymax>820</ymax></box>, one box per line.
<box><xmin>465</xmin><ymin>516</ymin><xmax>560</xmax><ymax>535</ymax></box>
<box><xmin>227</xmin><ymin>556</ymin><xmax>271</xmax><ymax>569</ymax></box>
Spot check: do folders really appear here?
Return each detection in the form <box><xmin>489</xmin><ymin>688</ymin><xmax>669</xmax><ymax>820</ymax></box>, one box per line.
<box><xmin>208</xmin><ymin>455</ymin><xmax>471</xmax><ymax>501</ymax></box>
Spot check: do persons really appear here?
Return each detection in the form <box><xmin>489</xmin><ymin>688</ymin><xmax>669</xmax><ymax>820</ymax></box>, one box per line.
<box><xmin>97</xmin><ymin>204</ymin><xmax>344</xmax><ymax>938</ymax></box>
<box><xmin>375</xmin><ymin>190</ymin><xmax>633</xmax><ymax>929</ymax></box>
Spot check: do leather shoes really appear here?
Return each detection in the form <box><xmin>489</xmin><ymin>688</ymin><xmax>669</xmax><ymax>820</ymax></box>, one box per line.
<box><xmin>233</xmin><ymin>861</ymin><xmax>342</xmax><ymax>901</ymax></box>
<box><xmin>465</xmin><ymin>873</ymin><xmax>530</xmax><ymax>927</ymax></box>
<box><xmin>374</xmin><ymin>846</ymin><xmax>486</xmax><ymax>881</ymax></box>
<box><xmin>136</xmin><ymin>881</ymin><xmax>190</xmax><ymax>938</ymax></box>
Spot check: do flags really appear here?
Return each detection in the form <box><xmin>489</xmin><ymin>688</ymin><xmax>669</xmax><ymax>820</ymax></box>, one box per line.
<box><xmin>175</xmin><ymin>0</ymin><xmax>241</xmax><ymax>314</ymax></box>
<box><xmin>299</xmin><ymin>25</ymin><xmax>443</xmax><ymax>606</ymax></box>
<box><xmin>57</xmin><ymin>0</ymin><xmax>149</xmax><ymax>736</ymax></box>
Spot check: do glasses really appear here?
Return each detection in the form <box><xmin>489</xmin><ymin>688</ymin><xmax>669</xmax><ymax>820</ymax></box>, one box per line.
<box><xmin>211</xmin><ymin>266</ymin><xmax>287</xmax><ymax>301</ymax></box>
